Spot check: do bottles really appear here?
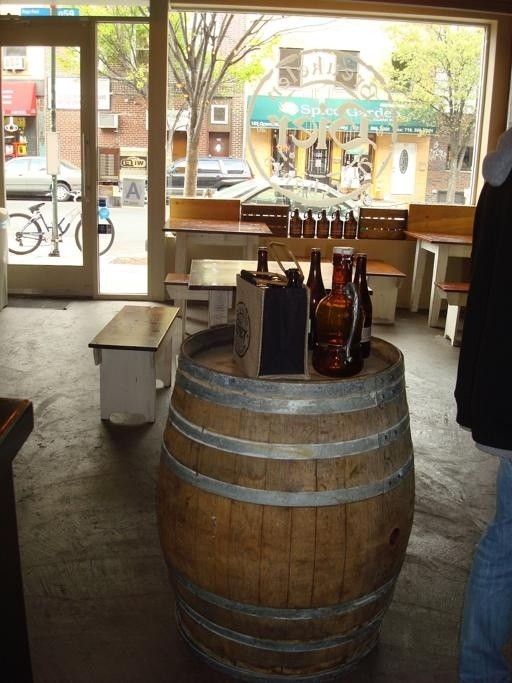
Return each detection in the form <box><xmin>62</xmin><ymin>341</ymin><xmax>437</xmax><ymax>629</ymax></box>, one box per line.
<box><xmin>311</xmin><ymin>246</ymin><xmax>364</xmax><ymax>378</ymax></box>
<box><xmin>306</xmin><ymin>247</ymin><xmax>326</xmax><ymax>350</ymax></box>
<box><xmin>286</xmin><ymin>268</ymin><xmax>302</xmax><ymax>289</ymax></box>
<box><xmin>290</xmin><ymin>207</ymin><xmax>357</xmax><ymax>239</ymax></box>
<box><xmin>353</xmin><ymin>252</ymin><xmax>372</xmax><ymax>358</ymax></box>
<box><xmin>256</xmin><ymin>245</ymin><xmax>269</xmax><ymax>272</ymax></box>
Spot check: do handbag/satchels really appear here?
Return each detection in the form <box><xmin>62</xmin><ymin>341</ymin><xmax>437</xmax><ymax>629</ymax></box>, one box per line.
<box><xmin>236</xmin><ymin>271</ymin><xmax>311</xmax><ymax>382</ymax></box>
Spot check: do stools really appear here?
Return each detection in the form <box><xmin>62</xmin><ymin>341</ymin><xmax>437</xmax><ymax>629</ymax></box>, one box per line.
<box><xmin>354</xmin><ymin>256</ymin><xmax>407</xmax><ymax>325</ymax></box>
<box><xmin>433</xmin><ymin>280</ymin><xmax>472</xmax><ymax>347</ymax></box>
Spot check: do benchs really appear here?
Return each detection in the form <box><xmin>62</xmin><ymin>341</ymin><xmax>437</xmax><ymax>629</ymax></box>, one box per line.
<box><xmin>166</xmin><ymin>272</ymin><xmax>233</xmax><ymax>344</ymax></box>
<box><xmin>88</xmin><ymin>304</ymin><xmax>182</xmax><ymax>424</ymax></box>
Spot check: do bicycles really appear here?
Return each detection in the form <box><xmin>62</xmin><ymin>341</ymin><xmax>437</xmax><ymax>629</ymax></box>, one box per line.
<box><xmin>5</xmin><ymin>188</ymin><xmax>120</xmax><ymax>256</ymax></box>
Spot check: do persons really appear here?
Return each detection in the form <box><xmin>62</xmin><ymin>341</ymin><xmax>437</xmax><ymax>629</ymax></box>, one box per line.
<box><xmin>453</xmin><ymin>119</ymin><xmax>511</xmax><ymax>683</ymax></box>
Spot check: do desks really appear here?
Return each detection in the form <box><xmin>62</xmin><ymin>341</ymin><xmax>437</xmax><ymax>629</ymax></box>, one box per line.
<box><xmin>405</xmin><ymin>229</ymin><xmax>474</xmax><ymax>326</ymax></box>
<box><xmin>0</xmin><ymin>395</ymin><xmax>38</xmax><ymax>682</ymax></box>
<box><xmin>187</xmin><ymin>257</ymin><xmax>373</xmax><ymax>327</ymax></box>
<box><xmin>162</xmin><ymin>217</ymin><xmax>272</xmax><ymax>314</ymax></box>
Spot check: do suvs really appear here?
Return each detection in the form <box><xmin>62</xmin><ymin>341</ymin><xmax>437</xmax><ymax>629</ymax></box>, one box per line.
<box><xmin>165</xmin><ymin>157</ymin><xmax>253</xmax><ymax>196</ymax></box>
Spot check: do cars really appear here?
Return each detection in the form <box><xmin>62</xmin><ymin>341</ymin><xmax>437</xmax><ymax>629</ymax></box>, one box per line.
<box><xmin>5</xmin><ymin>153</ymin><xmax>83</xmax><ymax>205</ymax></box>
<box><xmin>212</xmin><ymin>173</ymin><xmax>358</xmax><ymax>216</ymax></box>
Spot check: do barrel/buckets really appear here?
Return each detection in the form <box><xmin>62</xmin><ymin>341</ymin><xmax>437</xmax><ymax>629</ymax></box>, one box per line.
<box><xmin>157</xmin><ymin>326</ymin><xmax>417</xmax><ymax>682</ymax></box>
